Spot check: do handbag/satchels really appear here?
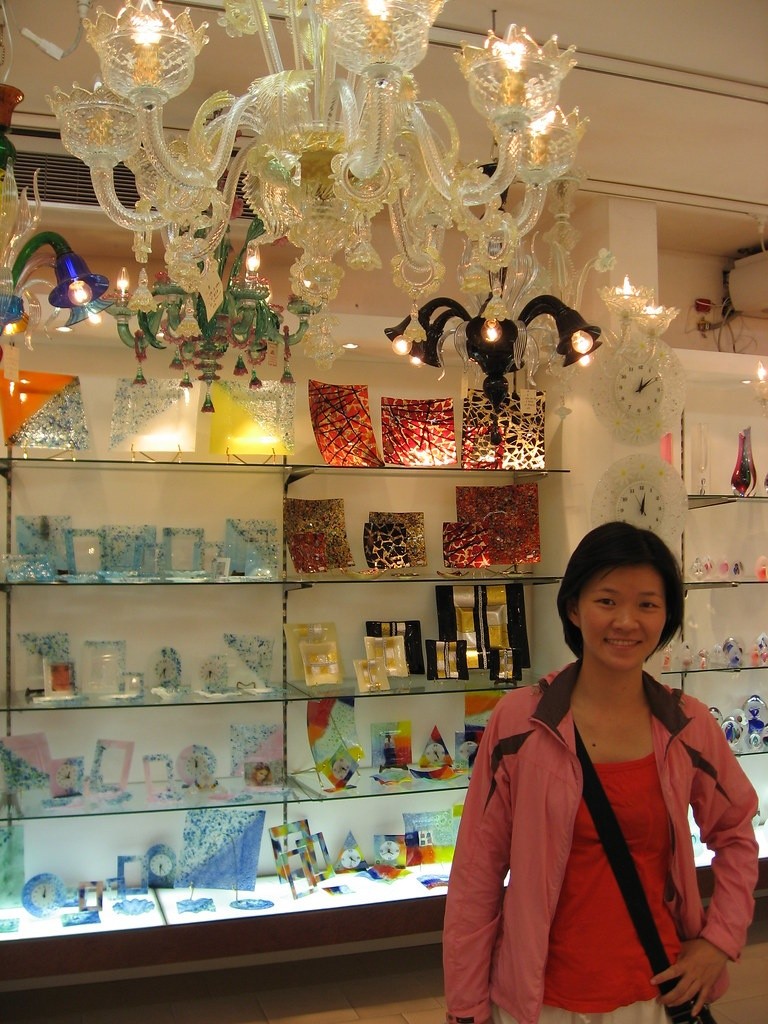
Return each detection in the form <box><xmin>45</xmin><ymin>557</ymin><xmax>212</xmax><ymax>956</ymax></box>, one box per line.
<box><xmin>672</xmin><ymin>992</ymin><xmax>717</xmax><ymax>1023</ymax></box>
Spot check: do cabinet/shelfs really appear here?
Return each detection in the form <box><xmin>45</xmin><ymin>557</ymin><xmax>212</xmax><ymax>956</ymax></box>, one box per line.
<box><xmin>675</xmin><ymin>404</ymin><xmax>768</xmax><ymax>871</ymax></box>
<box><xmin>1</xmin><ymin>442</ymin><xmax>571</xmax><ymax>1003</ymax></box>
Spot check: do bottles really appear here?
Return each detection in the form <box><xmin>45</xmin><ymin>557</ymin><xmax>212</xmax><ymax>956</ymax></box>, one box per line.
<box><xmin>684</xmin><ymin>418</ymin><xmax>759</xmax><ymax>500</ymax></box>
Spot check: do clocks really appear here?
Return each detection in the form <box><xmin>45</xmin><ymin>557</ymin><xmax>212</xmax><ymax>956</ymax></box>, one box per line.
<box><xmin>592</xmin><ymin>338</ymin><xmax>687</xmax><ymax>444</ymax></box>
<box><xmin>21</xmin><ymin>648</ymin><xmax>486</xmax><ymax>919</ymax></box>
<box><xmin>590</xmin><ymin>454</ymin><xmax>689</xmax><ymax>544</ymax></box>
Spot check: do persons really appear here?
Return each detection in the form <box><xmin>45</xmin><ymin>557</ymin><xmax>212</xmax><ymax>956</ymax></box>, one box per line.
<box><xmin>439</xmin><ymin>518</ymin><xmax>763</xmax><ymax>1024</ymax></box>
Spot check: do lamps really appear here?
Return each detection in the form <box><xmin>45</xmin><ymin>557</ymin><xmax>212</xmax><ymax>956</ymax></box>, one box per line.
<box><xmin>0</xmin><ymin>0</ymin><xmax>673</xmax><ymax>459</ymax></box>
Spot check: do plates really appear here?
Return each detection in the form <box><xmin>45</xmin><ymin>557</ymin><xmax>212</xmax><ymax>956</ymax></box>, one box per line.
<box><xmin>20</xmin><ymin>581</ymin><xmax>539</xmax><ymax>703</ymax></box>
<box><xmin>3</xmin><ymin>483</ymin><xmax>549</xmax><ymax>588</ymax></box>
<box><xmin>2</xmin><ymin>355</ymin><xmax>550</xmax><ymax>474</ymax></box>
